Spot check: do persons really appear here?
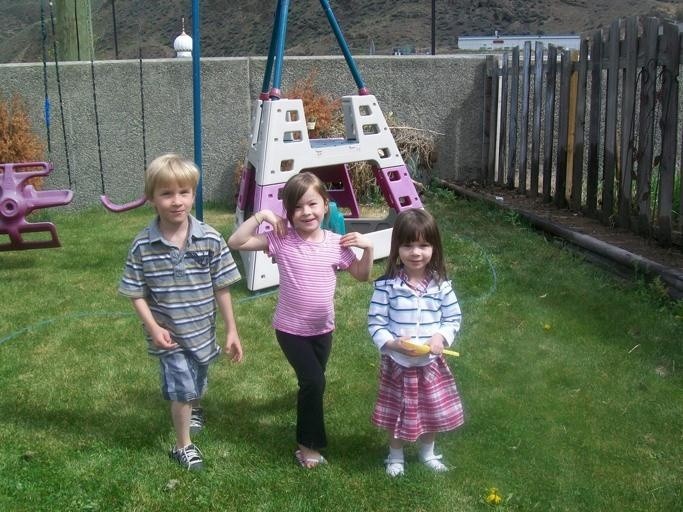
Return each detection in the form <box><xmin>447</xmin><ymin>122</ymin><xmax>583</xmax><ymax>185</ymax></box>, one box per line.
<box><xmin>115</xmin><ymin>153</ymin><xmax>244</xmax><ymax>475</ymax></box>
<box><xmin>227</xmin><ymin>172</ymin><xmax>373</xmax><ymax>470</ymax></box>
<box><xmin>363</xmin><ymin>205</ymin><xmax>464</xmax><ymax>478</ymax></box>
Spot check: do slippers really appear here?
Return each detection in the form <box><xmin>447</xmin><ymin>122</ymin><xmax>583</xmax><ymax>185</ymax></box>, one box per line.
<box><xmin>293</xmin><ymin>449</ymin><xmax>329</xmax><ymax>470</ymax></box>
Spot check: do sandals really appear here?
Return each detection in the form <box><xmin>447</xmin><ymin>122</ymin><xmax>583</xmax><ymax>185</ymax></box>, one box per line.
<box><xmin>419</xmin><ymin>453</ymin><xmax>449</xmax><ymax>473</ymax></box>
<box><xmin>383</xmin><ymin>453</ymin><xmax>405</xmax><ymax>478</ymax></box>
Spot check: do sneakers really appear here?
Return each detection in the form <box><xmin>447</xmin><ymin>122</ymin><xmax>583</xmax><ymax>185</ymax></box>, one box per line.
<box><xmin>188</xmin><ymin>406</ymin><xmax>203</xmax><ymax>433</ymax></box>
<box><xmin>171</xmin><ymin>442</ymin><xmax>204</xmax><ymax>471</ymax></box>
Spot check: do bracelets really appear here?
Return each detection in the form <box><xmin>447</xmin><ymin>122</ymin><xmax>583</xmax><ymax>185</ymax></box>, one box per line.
<box><xmin>253</xmin><ymin>212</ymin><xmax>260</xmax><ymax>226</ymax></box>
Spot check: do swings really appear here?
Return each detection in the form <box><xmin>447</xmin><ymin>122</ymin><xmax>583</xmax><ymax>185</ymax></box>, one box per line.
<box><xmin>40</xmin><ymin>0</ymin><xmax>74</xmax><ymax>206</ymax></box>
<box><xmin>87</xmin><ymin>1</ymin><xmax>148</xmax><ymax>213</ymax></box>
